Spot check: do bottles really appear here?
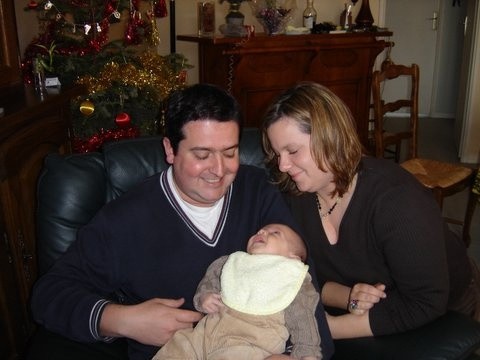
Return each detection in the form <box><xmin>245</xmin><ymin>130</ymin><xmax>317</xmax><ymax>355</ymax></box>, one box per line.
<box><xmin>340</xmin><ymin>3</ymin><xmax>353</xmax><ymax>30</ymax></box>
<box><xmin>303</xmin><ymin>0</ymin><xmax>317</xmax><ymax>29</ymax></box>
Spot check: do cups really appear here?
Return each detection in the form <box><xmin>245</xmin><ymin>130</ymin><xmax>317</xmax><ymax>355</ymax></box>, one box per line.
<box><xmin>33</xmin><ymin>57</ymin><xmax>46</xmax><ymax>95</ymax></box>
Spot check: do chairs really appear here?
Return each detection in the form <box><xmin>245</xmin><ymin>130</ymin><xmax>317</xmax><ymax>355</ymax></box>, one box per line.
<box><xmin>371</xmin><ymin>60</ymin><xmax>480</xmax><ymax>249</ymax></box>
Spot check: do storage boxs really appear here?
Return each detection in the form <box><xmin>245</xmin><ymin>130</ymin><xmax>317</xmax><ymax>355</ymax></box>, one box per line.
<box><xmin>0</xmin><ymin>65</ymin><xmax>26</xmax><ymax>110</ymax></box>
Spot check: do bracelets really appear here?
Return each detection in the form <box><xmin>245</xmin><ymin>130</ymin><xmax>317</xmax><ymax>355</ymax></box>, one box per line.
<box><xmin>346</xmin><ymin>288</ymin><xmax>352</xmax><ymax>311</ymax></box>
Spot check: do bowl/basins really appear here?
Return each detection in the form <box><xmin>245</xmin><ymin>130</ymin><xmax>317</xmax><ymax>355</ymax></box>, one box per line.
<box><xmin>219</xmin><ymin>23</ymin><xmax>251</xmax><ymax>38</ymax></box>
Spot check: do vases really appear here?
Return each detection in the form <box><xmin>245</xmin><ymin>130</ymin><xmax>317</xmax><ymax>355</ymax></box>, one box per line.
<box><xmin>356</xmin><ymin>0</ymin><xmax>375</xmax><ymax>28</ymax></box>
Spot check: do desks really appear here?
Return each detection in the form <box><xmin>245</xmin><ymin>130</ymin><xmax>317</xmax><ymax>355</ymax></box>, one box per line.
<box><xmin>176</xmin><ymin>26</ymin><xmax>394</xmax><ymax>150</ymax></box>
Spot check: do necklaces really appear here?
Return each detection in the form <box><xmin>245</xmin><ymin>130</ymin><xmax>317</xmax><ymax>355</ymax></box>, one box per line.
<box><xmin>316</xmin><ymin>194</ymin><xmax>341</xmax><ymax>218</ymax></box>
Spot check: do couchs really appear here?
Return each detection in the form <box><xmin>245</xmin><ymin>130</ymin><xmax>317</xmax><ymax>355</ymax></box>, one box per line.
<box><xmin>33</xmin><ymin>127</ymin><xmax>480</xmax><ymax>360</ymax></box>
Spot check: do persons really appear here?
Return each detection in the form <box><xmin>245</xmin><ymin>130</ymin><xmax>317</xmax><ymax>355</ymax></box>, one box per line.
<box><xmin>262</xmin><ymin>81</ymin><xmax>480</xmax><ymax>360</ymax></box>
<box><xmin>152</xmin><ymin>224</ymin><xmax>323</xmax><ymax>360</ymax></box>
<box><xmin>39</xmin><ymin>84</ymin><xmax>286</xmax><ymax>360</ymax></box>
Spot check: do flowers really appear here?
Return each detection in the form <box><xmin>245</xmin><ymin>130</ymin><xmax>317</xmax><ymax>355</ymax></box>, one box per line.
<box><xmin>255</xmin><ymin>5</ymin><xmax>292</xmax><ymax>38</ymax></box>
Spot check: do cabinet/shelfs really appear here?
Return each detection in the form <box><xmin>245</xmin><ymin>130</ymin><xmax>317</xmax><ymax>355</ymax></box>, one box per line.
<box><xmin>0</xmin><ymin>79</ymin><xmax>88</xmax><ymax>360</ymax></box>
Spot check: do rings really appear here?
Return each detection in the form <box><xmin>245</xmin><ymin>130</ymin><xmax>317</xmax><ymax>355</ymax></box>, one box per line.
<box><xmin>351</xmin><ymin>300</ymin><xmax>358</xmax><ymax>309</ymax></box>
